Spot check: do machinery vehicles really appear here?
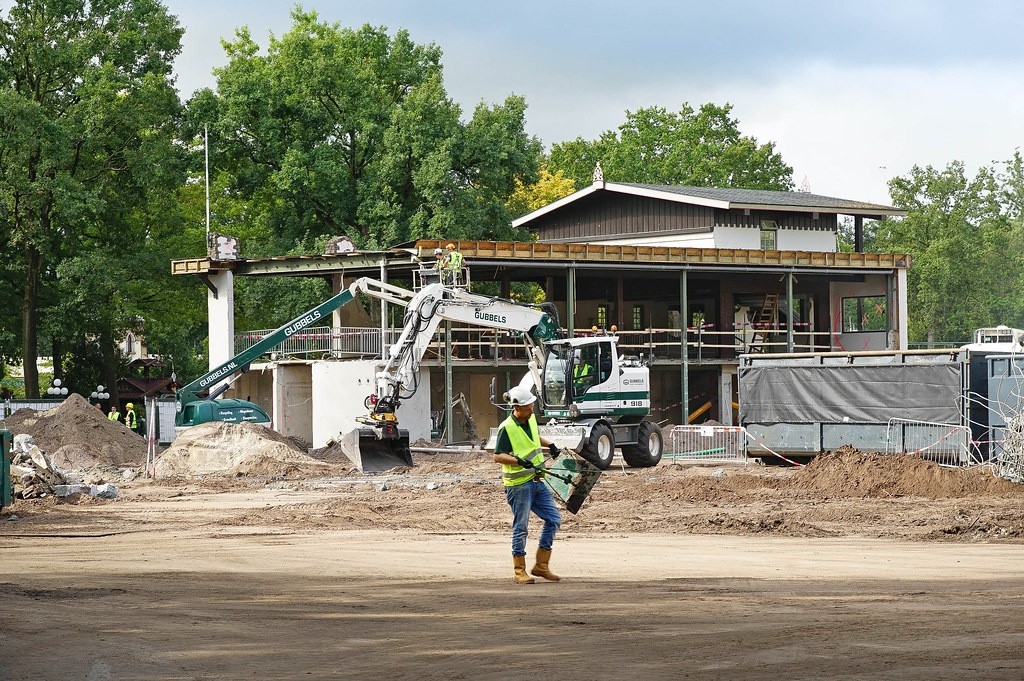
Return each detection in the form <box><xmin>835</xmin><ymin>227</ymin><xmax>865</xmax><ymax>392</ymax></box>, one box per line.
<box><xmin>173</xmin><ymin>277</ymin><xmax>420</xmax><ymax>443</ymax></box>
<box><xmin>341</xmin><ymin>282</ymin><xmax>663</xmax><ymax>480</ymax></box>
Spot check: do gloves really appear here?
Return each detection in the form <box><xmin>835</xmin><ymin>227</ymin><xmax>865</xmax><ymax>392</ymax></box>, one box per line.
<box><xmin>547</xmin><ymin>443</ymin><xmax>560</xmax><ymax>459</ymax></box>
<box><xmin>518</xmin><ymin>458</ymin><xmax>534</xmax><ymax>470</ymax></box>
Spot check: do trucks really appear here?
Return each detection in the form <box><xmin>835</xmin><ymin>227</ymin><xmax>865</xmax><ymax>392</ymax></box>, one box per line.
<box><xmin>739</xmin><ymin>326</ymin><xmax>1024</xmax><ymax>485</ymax></box>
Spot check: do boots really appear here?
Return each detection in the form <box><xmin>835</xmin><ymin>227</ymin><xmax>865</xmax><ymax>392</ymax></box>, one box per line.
<box><xmin>530</xmin><ymin>547</ymin><xmax>560</xmax><ymax>582</ymax></box>
<box><xmin>513</xmin><ymin>554</ymin><xmax>535</xmax><ymax>584</ymax></box>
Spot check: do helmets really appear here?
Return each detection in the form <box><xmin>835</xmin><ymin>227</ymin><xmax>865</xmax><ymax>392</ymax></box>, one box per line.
<box><xmin>434</xmin><ymin>248</ymin><xmax>444</xmax><ymax>255</ymax></box>
<box><xmin>507</xmin><ymin>386</ymin><xmax>537</xmax><ymax>406</ymax></box>
<box><xmin>446</xmin><ymin>243</ymin><xmax>456</xmax><ymax>251</ymax></box>
<box><xmin>125</xmin><ymin>403</ymin><xmax>134</xmax><ymax>410</ymax></box>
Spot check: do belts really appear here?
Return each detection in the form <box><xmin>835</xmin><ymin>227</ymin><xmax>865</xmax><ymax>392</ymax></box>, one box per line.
<box><xmin>532</xmin><ymin>475</ymin><xmax>541</xmax><ymax>483</ymax></box>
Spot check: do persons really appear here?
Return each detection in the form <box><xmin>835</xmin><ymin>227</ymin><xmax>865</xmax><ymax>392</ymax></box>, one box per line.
<box><xmin>436</xmin><ymin>243</ymin><xmax>466</xmax><ymax>288</ymax></box>
<box><xmin>125</xmin><ymin>402</ymin><xmax>138</xmax><ymax>433</ymax></box>
<box><xmin>94</xmin><ymin>403</ymin><xmax>102</xmax><ymax>409</ymax></box>
<box><xmin>432</xmin><ymin>248</ymin><xmax>451</xmax><ymax>287</ymax></box>
<box><xmin>107</xmin><ymin>405</ymin><xmax>123</xmax><ymax>423</ymax></box>
<box><xmin>558</xmin><ymin>353</ymin><xmax>595</xmax><ymax>405</ymax></box>
<box><xmin>492</xmin><ymin>385</ymin><xmax>563</xmax><ymax>585</ymax></box>
